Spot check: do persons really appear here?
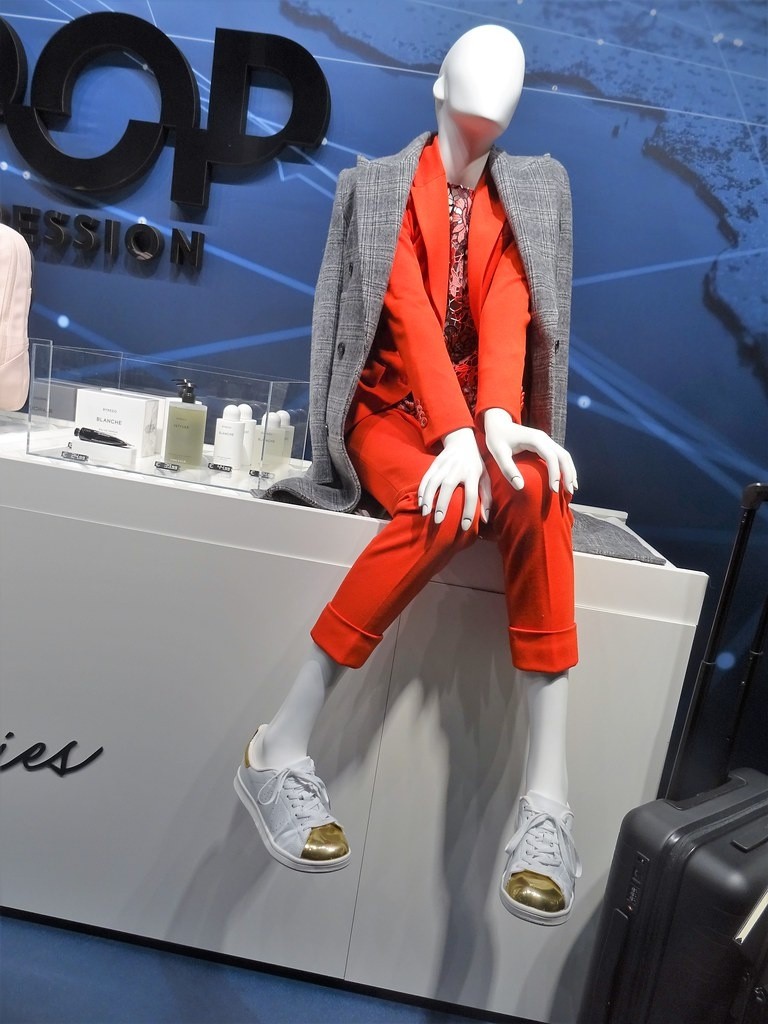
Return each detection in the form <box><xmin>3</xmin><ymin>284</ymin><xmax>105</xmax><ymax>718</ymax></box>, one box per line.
<box><xmin>233</xmin><ymin>24</ymin><xmax>578</xmax><ymax>926</ymax></box>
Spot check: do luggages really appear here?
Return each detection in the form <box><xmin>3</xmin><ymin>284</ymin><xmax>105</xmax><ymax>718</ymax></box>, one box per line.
<box><xmin>578</xmin><ymin>485</ymin><xmax>768</xmax><ymax>1022</ymax></box>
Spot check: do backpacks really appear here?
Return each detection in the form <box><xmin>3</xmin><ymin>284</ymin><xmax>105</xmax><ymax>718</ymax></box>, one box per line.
<box><xmin>0</xmin><ymin>222</ymin><xmax>38</xmax><ymax>414</ymax></box>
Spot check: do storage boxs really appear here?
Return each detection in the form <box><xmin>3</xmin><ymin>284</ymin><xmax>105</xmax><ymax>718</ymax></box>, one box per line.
<box><xmin>101</xmin><ymin>388</ymin><xmax>167</xmax><ymax>454</ymax></box>
<box><xmin>26</xmin><ymin>344</ymin><xmax>310</xmax><ymax>492</ymax></box>
<box><xmin>31</xmin><ymin>381</ymin><xmax>86</xmax><ymax>422</ymax></box>
<box><xmin>75</xmin><ymin>388</ymin><xmax>159</xmax><ymax>458</ymax></box>
<box><xmin>37</xmin><ymin>377</ymin><xmax>109</xmax><ymax>388</ymax></box>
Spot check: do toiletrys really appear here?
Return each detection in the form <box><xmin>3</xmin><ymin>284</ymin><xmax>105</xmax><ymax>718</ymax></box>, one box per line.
<box><xmin>163</xmin><ymin>377</ymin><xmax>294</xmax><ymax>471</ymax></box>
<box><xmin>74</xmin><ymin>427</ymin><xmax>134</xmax><ymax>450</ymax></box>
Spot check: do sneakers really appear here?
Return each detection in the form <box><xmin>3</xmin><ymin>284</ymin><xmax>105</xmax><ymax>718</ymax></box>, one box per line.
<box><xmin>226</xmin><ymin>724</ymin><xmax>351</xmax><ymax>874</ymax></box>
<box><xmin>498</xmin><ymin>790</ymin><xmax>583</xmax><ymax>928</ymax></box>
